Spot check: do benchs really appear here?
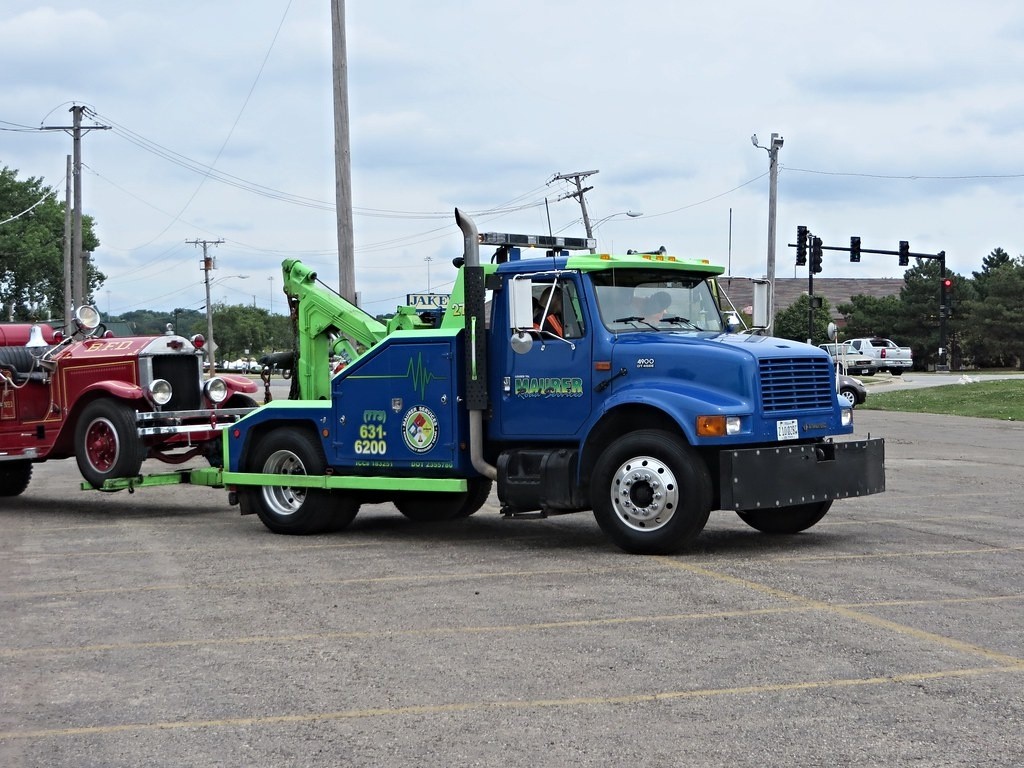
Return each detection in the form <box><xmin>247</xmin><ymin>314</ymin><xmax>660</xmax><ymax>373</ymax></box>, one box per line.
<box><xmin>0</xmin><ymin>344</ymin><xmax>66</xmax><ymax>380</ymax></box>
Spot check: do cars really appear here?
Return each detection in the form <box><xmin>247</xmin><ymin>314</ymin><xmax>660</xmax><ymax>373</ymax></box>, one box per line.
<box><xmin>818</xmin><ymin>343</ymin><xmax>877</xmax><ymax>375</ymax></box>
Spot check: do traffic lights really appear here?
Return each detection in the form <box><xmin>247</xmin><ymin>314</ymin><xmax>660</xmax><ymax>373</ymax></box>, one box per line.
<box><xmin>899</xmin><ymin>240</ymin><xmax>909</xmax><ymax>267</ymax></box>
<box><xmin>849</xmin><ymin>237</ymin><xmax>861</xmax><ymax>262</ymax></box>
<box><xmin>945</xmin><ymin>279</ymin><xmax>952</xmax><ymax>294</ymax></box>
<box><xmin>810</xmin><ymin>297</ymin><xmax>823</xmax><ymax>309</ymax></box>
<box><xmin>812</xmin><ymin>237</ymin><xmax>823</xmax><ymax>274</ymax></box>
<box><xmin>796</xmin><ymin>226</ymin><xmax>809</xmax><ymax>266</ymax></box>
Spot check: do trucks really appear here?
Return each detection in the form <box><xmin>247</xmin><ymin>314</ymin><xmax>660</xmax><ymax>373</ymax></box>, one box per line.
<box><xmin>78</xmin><ymin>208</ymin><xmax>886</xmax><ymax>555</ymax></box>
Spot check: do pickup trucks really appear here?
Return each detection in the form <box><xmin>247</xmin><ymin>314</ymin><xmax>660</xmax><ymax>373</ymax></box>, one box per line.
<box><xmin>843</xmin><ymin>337</ymin><xmax>913</xmax><ymax>376</ymax></box>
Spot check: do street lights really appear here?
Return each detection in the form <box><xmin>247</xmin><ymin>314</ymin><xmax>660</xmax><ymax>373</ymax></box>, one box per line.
<box><xmin>587</xmin><ymin>209</ymin><xmax>645</xmax><ymax>255</ymax></box>
<box><xmin>750</xmin><ymin>131</ymin><xmax>785</xmax><ymax>338</ymax></box>
<box><xmin>267</xmin><ymin>276</ymin><xmax>274</xmax><ymax>316</ymax></box>
<box><xmin>423</xmin><ymin>256</ymin><xmax>435</xmax><ymax>294</ymax></box>
<box><xmin>206</xmin><ymin>275</ymin><xmax>250</xmax><ymax>379</ymax></box>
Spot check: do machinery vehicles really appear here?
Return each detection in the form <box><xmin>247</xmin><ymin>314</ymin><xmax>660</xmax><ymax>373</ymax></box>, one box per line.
<box><xmin>0</xmin><ymin>305</ymin><xmax>261</xmax><ymax>498</ymax></box>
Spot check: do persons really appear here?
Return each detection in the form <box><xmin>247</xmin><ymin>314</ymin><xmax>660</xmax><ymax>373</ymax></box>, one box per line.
<box><xmin>523</xmin><ymin>285</ymin><xmax>563</xmax><ymax>341</ymax></box>
<box><xmin>629</xmin><ymin>291</ymin><xmax>672</xmax><ymax>323</ymax></box>
